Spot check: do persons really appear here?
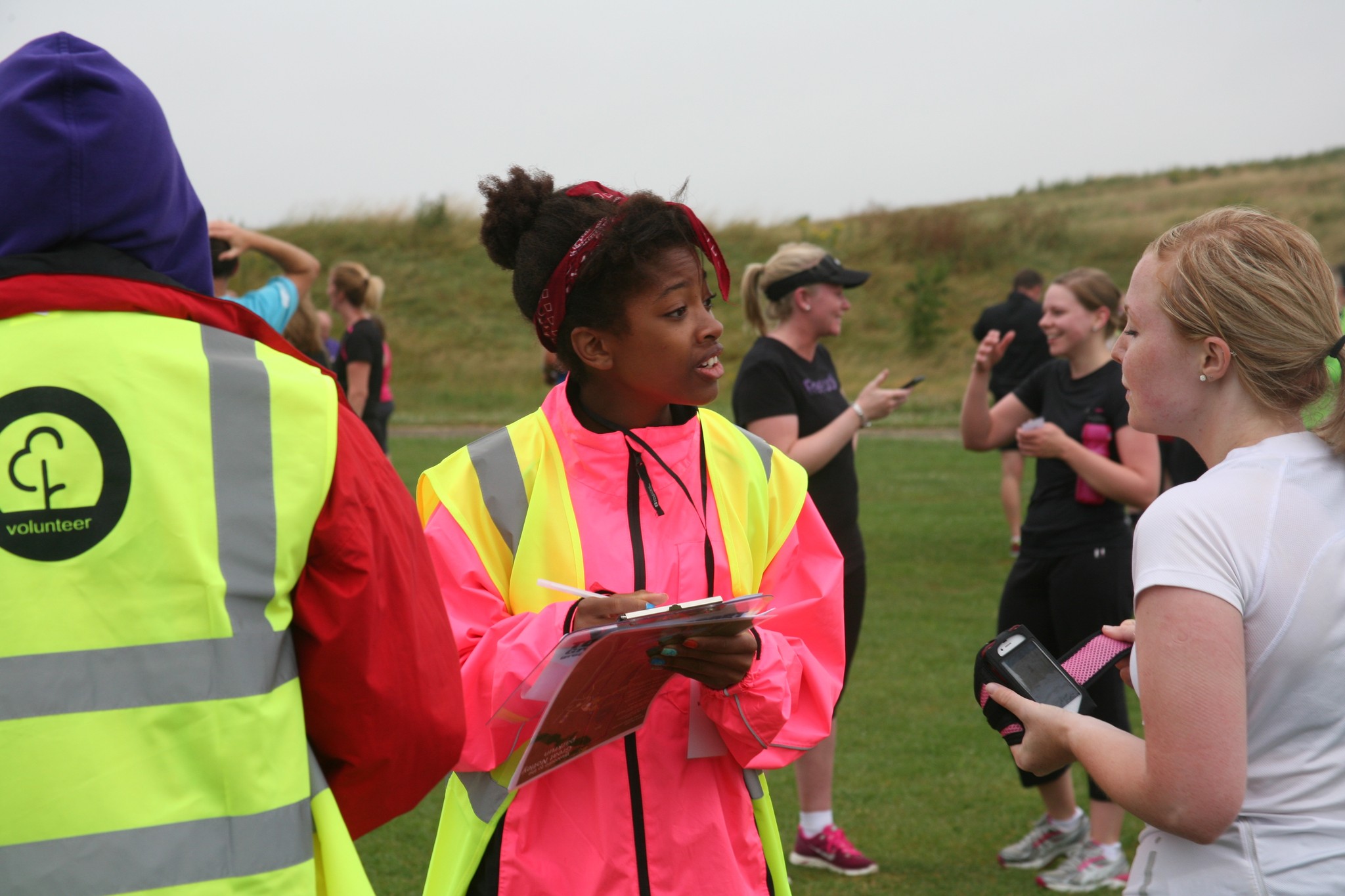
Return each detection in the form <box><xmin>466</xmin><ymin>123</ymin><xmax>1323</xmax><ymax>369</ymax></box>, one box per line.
<box><xmin>378</xmin><ymin>340</ymin><xmax>395</xmax><ymax>462</ymax></box>
<box><xmin>730</xmin><ymin>239</ymin><xmax>909</xmax><ymax>878</ymax></box>
<box><xmin>957</xmin><ymin>266</ymin><xmax>1164</xmax><ymax>893</ymax></box>
<box><xmin>283</xmin><ymin>294</ymin><xmax>340</xmax><ymax>371</ymax></box>
<box><xmin>969</xmin><ymin>268</ymin><xmax>1049</xmax><ymax>552</ymax></box>
<box><xmin>325</xmin><ymin>261</ymin><xmax>387</xmax><ymax>445</ymax></box>
<box><xmin>0</xmin><ymin>28</ymin><xmax>470</xmax><ymax>896</ymax></box>
<box><xmin>418</xmin><ymin>167</ymin><xmax>852</xmax><ymax>896</ymax></box>
<box><xmin>207</xmin><ymin>218</ymin><xmax>322</xmax><ymax>337</ymax></box>
<box><xmin>982</xmin><ymin>203</ymin><xmax>1343</xmax><ymax>896</ymax></box>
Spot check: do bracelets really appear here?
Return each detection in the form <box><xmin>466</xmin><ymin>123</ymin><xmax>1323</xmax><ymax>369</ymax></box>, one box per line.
<box><xmin>850</xmin><ymin>402</ymin><xmax>867</xmax><ymax>429</ymax></box>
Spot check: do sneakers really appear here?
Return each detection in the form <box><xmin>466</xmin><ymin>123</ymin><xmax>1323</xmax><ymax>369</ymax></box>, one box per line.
<box><xmin>790</xmin><ymin>824</ymin><xmax>880</xmax><ymax>875</ymax></box>
<box><xmin>999</xmin><ymin>805</ymin><xmax>1091</xmax><ymax>870</ymax></box>
<box><xmin>1035</xmin><ymin>835</ymin><xmax>1130</xmax><ymax>892</ymax></box>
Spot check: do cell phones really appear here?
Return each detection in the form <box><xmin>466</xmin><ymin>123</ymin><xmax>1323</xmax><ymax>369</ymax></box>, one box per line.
<box><xmin>899</xmin><ymin>376</ymin><xmax>925</xmax><ymax>390</ymax></box>
<box><xmin>985</xmin><ymin>624</ymin><xmax>1098</xmax><ymax>717</ymax></box>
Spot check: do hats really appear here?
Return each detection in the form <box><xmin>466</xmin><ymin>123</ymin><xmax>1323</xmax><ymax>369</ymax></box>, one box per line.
<box><xmin>762</xmin><ymin>255</ymin><xmax>870</xmax><ymax>305</ymax></box>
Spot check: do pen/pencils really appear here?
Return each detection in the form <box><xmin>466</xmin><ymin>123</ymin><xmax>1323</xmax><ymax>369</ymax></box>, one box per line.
<box><xmin>536</xmin><ymin>577</ymin><xmax>609</xmax><ymax>599</ymax></box>
